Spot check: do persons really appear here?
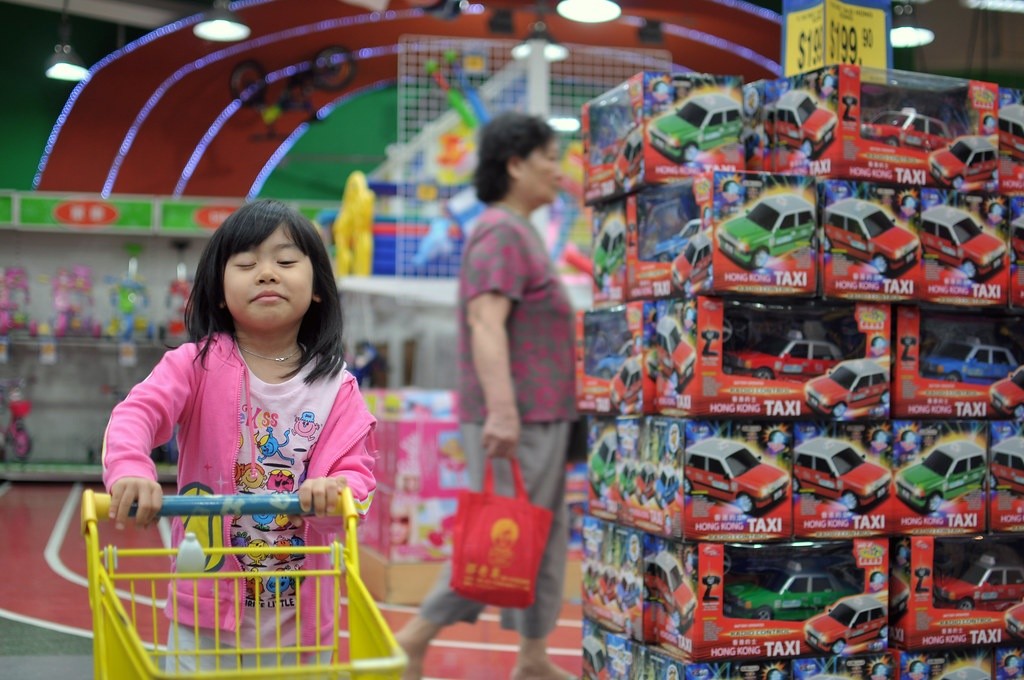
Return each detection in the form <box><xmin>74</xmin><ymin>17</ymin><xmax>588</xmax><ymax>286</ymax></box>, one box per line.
<box><xmin>101</xmin><ymin>201</ymin><xmax>380</xmax><ymax>676</ymax></box>
<box><xmin>395</xmin><ymin>112</ymin><xmax>580</xmax><ymax>680</ymax></box>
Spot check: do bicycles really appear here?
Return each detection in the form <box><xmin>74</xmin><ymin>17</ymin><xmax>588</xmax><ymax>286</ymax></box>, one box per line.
<box><xmin>226</xmin><ymin>45</ymin><xmax>366</xmax><ymax>142</ymax></box>
<box><xmin>0</xmin><ymin>377</ymin><xmax>45</xmax><ymax>468</ymax></box>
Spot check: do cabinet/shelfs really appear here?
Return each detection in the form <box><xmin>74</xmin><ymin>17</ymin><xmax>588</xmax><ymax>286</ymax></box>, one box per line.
<box><xmin>1</xmin><ymin>190</ymin><xmax>336</xmax><ymax>489</ymax></box>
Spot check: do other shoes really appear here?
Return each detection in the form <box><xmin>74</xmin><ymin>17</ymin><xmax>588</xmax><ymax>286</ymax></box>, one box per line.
<box><xmin>507</xmin><ymin>668</ymin><xmax>577</xmax><ymax>680</ymax></box>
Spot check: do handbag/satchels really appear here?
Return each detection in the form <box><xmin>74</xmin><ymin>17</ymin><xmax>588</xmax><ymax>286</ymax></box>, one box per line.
<box><xmin>450</xmin><ymin>452</ymin><xmax>555</xmax><ymax>609</ymax></box>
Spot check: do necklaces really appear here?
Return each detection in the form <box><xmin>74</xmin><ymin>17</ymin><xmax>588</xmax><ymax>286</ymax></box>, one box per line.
<box><xmin>238</xmin><ymin>344</ymin><xmax>300</xmax><ymax>362</ymax></box>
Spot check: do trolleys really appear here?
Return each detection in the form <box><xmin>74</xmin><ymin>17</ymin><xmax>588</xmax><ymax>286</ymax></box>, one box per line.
<box><xmin>78</xmin><ymin>480</ymin><xmax>413</xmax><ymax>680</ymax></box>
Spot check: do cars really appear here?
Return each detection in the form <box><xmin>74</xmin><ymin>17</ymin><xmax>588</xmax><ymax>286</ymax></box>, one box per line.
<box><xmin>576</xmin><ymin>67</ymin><xmax>1024</xmax><ymax>679</ymax></box>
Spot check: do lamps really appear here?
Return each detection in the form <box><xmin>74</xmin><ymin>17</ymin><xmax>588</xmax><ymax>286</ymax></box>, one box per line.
<box><xmin>890</xmin><ymin>0</ymin><xmax>936</xmax><ymax>48</ymax></box>
<box><xmin>511</xmin><ymin>0</ymin><xmax>568</xmax><ymax>62</ymax></box>
<box><xmin>554</xmin><ymin>0</ymin><xmax>621</xmax><ymax>25</ymax></box>
<box><xmin>193</xmin><ymin>0</ymin><xmax>251</xmax><ymax>42</ymax></box>
<box><xmin>41</xmin><ymin>0</ymin><xmax>89</xmax><ymax>81</ymax></box>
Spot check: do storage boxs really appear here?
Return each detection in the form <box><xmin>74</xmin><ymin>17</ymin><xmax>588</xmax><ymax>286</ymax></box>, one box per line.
<box><xmin>353</xmin><ymin>388</ymin><xmax>472</xmax><ymax>568</ymax></box>
<box><xmin>565</xmin><ymin>64</ymin><xmax>1023</xmax><ymax>679</ymax></box>
